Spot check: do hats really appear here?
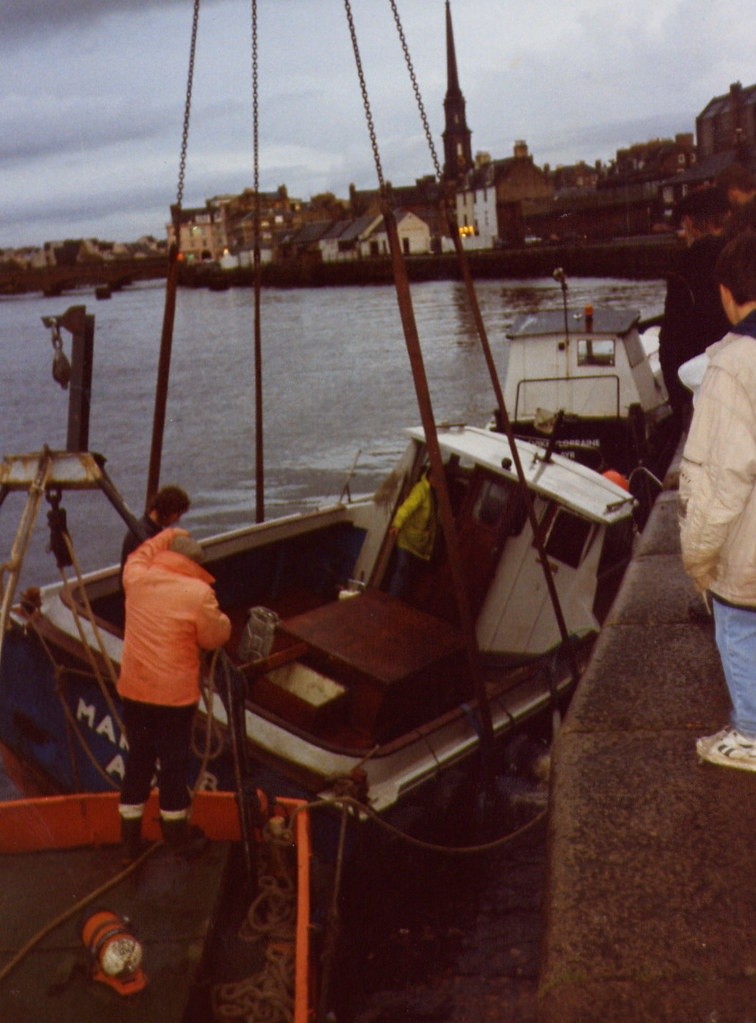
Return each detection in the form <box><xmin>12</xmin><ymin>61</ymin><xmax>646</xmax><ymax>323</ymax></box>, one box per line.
<box><xmin>172</xmin><ymin>535</ymin><xmax>206</xmax><ymax>565</ymax></box>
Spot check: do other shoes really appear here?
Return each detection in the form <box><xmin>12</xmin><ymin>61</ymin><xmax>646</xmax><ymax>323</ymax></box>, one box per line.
<box><xmin>119</xmin><ymin>804</ymin><xmax>144</xmax><ymax>818</ymax></box>
<box><xmin>160</xmin><ymin>809</ymin><xmax>186</xmax><ymax>821</ymax></box>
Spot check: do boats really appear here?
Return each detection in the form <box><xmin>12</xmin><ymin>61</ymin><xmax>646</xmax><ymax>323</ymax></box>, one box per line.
<box><xmin>0</xmin><ymin>302</ymin><xmax>681</xmax><ymax>907</ymax></box>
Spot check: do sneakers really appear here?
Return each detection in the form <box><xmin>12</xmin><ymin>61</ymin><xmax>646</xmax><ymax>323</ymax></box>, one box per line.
<box><xmin>697</xmin><ymin>725</ymin><xmax>756</xmax><ymax>773</ymax></box>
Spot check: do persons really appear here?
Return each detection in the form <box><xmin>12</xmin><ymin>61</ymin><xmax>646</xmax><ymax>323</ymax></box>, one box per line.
<box><xmin>384</xmin><ymin>466</ymin><xmax>440</xmax><ymax>596</ymax></box>
<box><xmin>720</xmin><ymin>161</ymin><xmax>756</xmax><ymax>227</ymax></box>
<box><xmin>660</xmin><ymin>185</ymin><xmax>733</xmax><ymax>435</ymax></box>
<box><xmin>677</xmin><ymin>231</ymin><xmax>756</xmax><ymax>775</ymax></box>
<box><xmin>115</xmin><ymin>526</ymin><xmax>230</xmax><ymax>821</ymax></box>
<box><xmin>120</xmin><ymin>485</ymin><xmax>189</xmax><ymax>609</ymax></box>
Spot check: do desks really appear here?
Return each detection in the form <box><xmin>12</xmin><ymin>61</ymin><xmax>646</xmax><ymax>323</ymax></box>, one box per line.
<box><xmin>284</xmin><ymin>588</ymin><xmax>469</xmax><ymax>753</ymax></box>
